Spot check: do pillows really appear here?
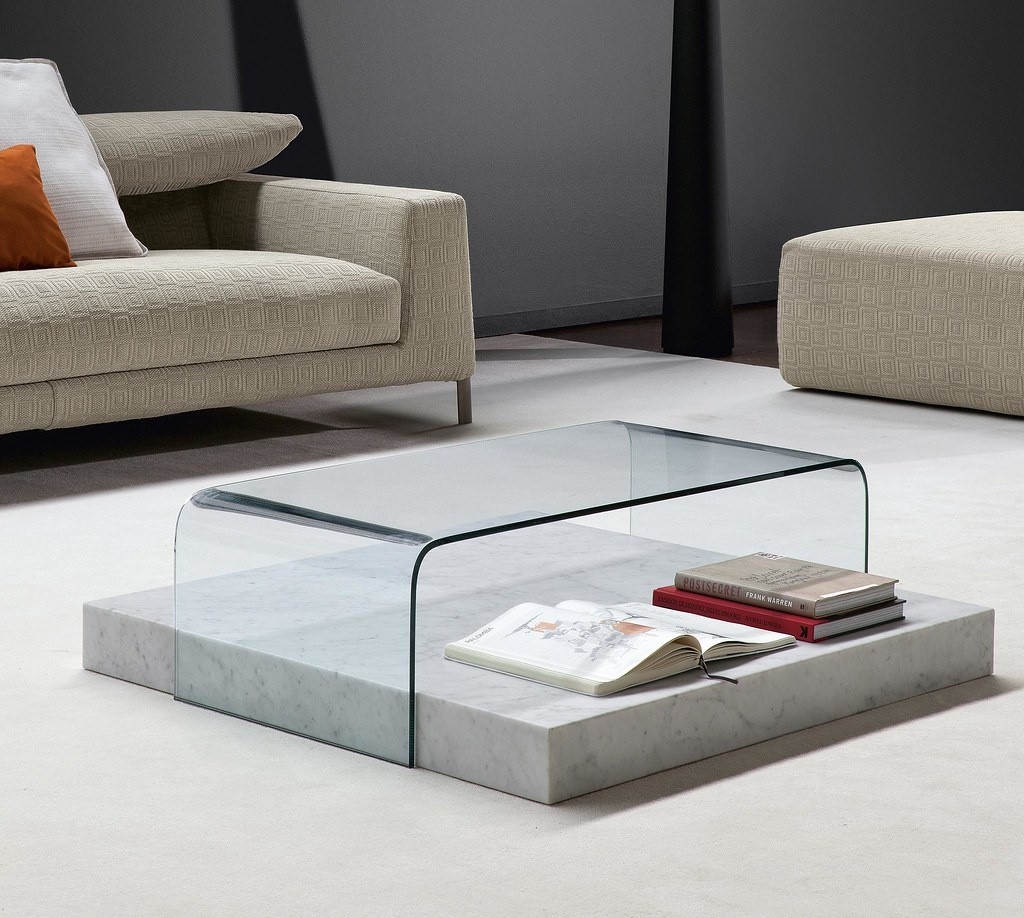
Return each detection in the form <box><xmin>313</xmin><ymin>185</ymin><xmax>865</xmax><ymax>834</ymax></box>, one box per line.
<box><xmin>0</xmin><ymin>145</ymin><xmax>78</xmax><ymax>273</ymax></box>
<box><xmin>0</xmin><ymin>58</ymin><xmax>148</xmax><ymax>259</ymax></box>
<box><xmin>77</xmin><ymin>110</ymin><xmax>303</xmax><ymax>197</ymax></box>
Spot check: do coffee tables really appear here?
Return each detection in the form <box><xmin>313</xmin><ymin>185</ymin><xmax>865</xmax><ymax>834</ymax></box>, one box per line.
<box><xmin>83</xmin><ymin>420</ymin><xmax>998</xmax><ymax>806</ymax></box>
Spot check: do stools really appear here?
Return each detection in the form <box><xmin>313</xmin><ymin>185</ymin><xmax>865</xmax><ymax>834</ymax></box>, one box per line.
<box><xmin>776</xmin><ymin>211</ymin><xmax>1024</xmax><ymax>420</ymax></box>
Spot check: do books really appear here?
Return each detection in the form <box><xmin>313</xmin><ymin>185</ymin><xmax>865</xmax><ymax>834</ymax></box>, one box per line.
<box><xmin>444</xmin><ymin>600</ymin><xmax>797</xmax><ymax>698</ymax></box>
<box><xmin>652</xmin><ymin>585</ymin><xmax>907</xmax><ymax>644</ymax></box>
<box><xmin>675</xmin><ymin>551</ymin><xmax>899</xmax><ymax>619</ymax></box>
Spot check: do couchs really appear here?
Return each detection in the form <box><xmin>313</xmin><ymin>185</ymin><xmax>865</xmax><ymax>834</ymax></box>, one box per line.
<box><xmin>0</xmin><ymin>172</ymin><xmax>476</xmax><ymax>437</ymax></box>
